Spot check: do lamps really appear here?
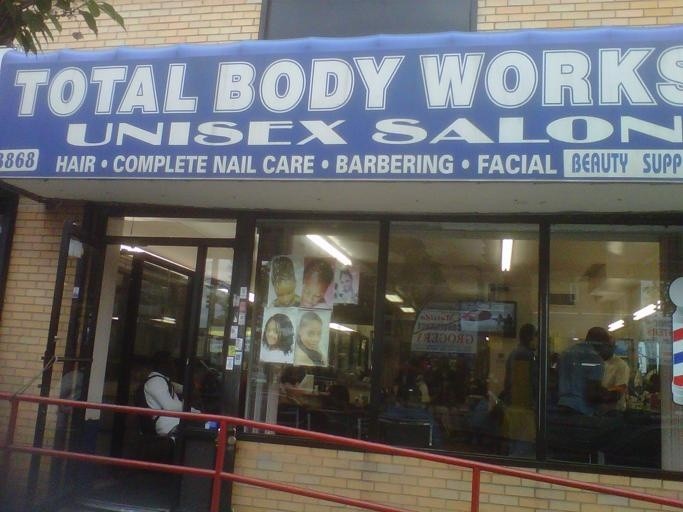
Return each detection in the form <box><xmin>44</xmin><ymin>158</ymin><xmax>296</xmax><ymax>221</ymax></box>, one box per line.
<box><xmin>383</xmin><ymin>291</ymin><xmax>417</xmax><ymax>314</ymax></box>
<box><xmin>500</xmin><ymin>238</ymin><xmax>513</xmax><ymax>274</ymax></box>
<box><xmin>306</xmin><ymin>233</ymin><xmax>352</xmax><ymax>266</ymax></box>
<box><xmin>608</xmin><ymin>302</ymin><xmax>657</xmax><ymax>332</ymax></box>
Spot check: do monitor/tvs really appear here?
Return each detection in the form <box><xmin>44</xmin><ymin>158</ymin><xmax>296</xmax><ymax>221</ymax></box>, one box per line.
<box><xmin>457</xmin><ymin>300</ymin><xmax>517</xmax><ymax>339</ymax></box>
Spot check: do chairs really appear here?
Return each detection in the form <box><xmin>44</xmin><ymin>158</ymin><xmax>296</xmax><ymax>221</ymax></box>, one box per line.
<box><xmin>133</xmin><ymin>388</ymin><xmax>178</xmax><ymax>473</ymax></box>
<box><xmin>325</xmin><ymin>384</ymin><xmax>357</xmax><ymax>409</ymax></box>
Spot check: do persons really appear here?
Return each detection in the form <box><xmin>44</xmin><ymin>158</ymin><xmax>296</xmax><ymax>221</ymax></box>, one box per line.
<box><xmin>143</xmin><ymin>323</ymin><xmax>660</xmax><ymax>463</ymax></box>
<box><xmin>57</xmin><ymin>363</ymin><xmax>85</xmax><ymax>428</ymax></box>
<box><xmin>261</xmin><ymin>256</ymin><xmax>357</xmax><ymax>366</ymax></box>
<box><xmin>470</xmin><ymin>311</ymin><xmax>502</xmax><ymax>330</ymax></box>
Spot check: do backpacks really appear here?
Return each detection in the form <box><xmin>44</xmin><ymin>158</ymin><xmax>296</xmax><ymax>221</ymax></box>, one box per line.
<box><xmin>134</xmin><ymin>375</ymin><xmax>173</xmax><ymax>439</ymax></box>
